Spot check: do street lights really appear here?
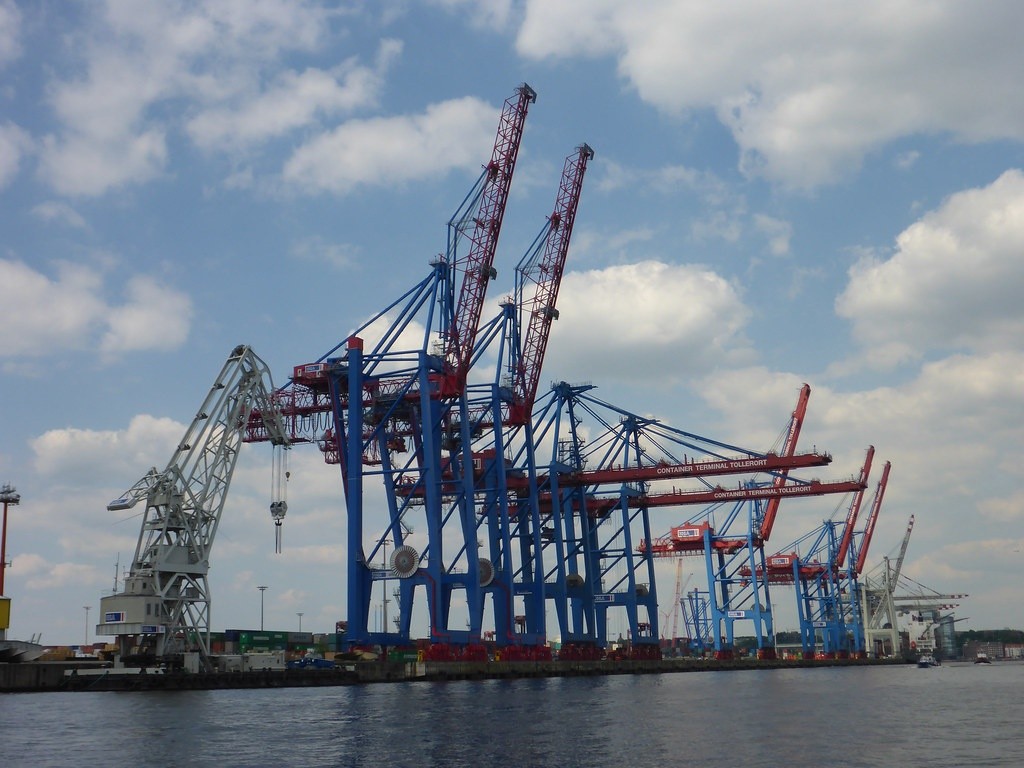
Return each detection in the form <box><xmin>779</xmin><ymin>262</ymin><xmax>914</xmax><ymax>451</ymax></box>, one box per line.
<box><xmin>297</xmin><ymin>612</ymin><xmax>303</xmax><ymax>631</ymax></box>
<box><xmin>257</xmin><ymin>586</ymin><xmax>268</xmax><ymax>631</ymax></box>
<box><xmin>376</xmin><ymin>539</ymin><xmax>393</xmax><ymax>632</ymax></box>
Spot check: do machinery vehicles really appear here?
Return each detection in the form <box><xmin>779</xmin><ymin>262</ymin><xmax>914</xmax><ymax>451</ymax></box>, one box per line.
<box><xmin>63</xmin><ymin>344</ymin><xmax>294</xmax><ymax>675</ymax></box>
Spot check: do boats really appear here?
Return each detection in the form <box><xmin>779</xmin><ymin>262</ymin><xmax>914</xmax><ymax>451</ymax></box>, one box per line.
<box><xmin>920</xmin><ymin>656</ymin><xmax>942</xmax><ymax>668</ymax></box>
<box><xmin>975</xmin><ymin>656</ymin><xmax>990</xmax><ymax>665</ymax></box>
